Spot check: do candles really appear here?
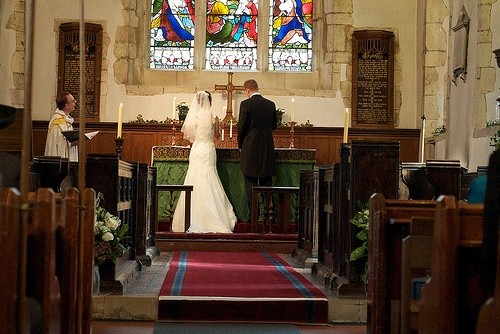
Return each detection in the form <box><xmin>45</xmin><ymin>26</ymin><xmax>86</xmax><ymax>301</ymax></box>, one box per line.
<box><xmin>342</xmin><ymin>108</ymin><xmax>349</xmax><ymax>143</ymax></box>
<box><xmin>230</xmin><ymin>120</ymin><xmax>232</xmax><ymax>139</ymax></box>
<box><xmin>290</xmin><ymin>98</ymin><xmax>295</xmax><ymax>121</ymax></box>
<box><xmin>117</xmin><ymin>103</ymin><xmax>122</xmax><ymax>138</ymax></box>
<box><xmin>418</xmin><ymin>119</ymin><xmax>425</xmax><ymax>163</ymax></box>
<box><xmin>173</xmin><ymin>97</ymin><xmax>176</xmax><ymax>119</ymax></box>
<box><xmin>221</xmin><ymin>128</ymin><xmax>224</xmax><ymax>141</ymax></box>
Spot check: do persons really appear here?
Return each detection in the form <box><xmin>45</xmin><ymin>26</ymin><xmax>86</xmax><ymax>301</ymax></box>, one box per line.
<box><xmin>237</xmin><ymin>80</ymin><xmax>278</xmax><ymax>223</ymax></box>
<box><xmin>45</xmin><ymin>91</ymin><xmax>78</xmax><ymax>162</ymax></box>
<box><xmin>171</xmin><ymin>91</ymin><xmax>238</xmax><ymax>234</ymax></box>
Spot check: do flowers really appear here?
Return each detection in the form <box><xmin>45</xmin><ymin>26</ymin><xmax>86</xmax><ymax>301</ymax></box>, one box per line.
<box><xmin>94</xmin><ymin>206</ymin><xmax>131</xmax><ymax>263</ymax></box>
<box><xmin>347</xmin><ymin>197</ymin><xmax>370</xmax><ymax>283</ymax></box>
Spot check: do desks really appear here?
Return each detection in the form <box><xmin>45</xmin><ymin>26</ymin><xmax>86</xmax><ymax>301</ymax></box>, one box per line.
<box><xmin>157</xmin><ymin>184</ymin><xmax>193</xmax><ymax>234</ymax></box>
<box><xmin>250</xmin><ymin>186</ymin><xmax>300</xmax><ymax>234</ymax></box>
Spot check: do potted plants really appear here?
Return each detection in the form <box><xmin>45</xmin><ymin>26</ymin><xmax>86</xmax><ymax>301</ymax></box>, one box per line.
<box><xmin>176</xmin><ymin>102</ymin><xmax>190</xmax><ymax>120</ymax></box>
<box><xmin>276</xmin><ymin>108</ymin><xmax>285</xmax><ymax>122</ymax></box>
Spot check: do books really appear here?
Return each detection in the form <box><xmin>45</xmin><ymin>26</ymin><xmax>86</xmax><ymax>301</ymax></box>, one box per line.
<box><xmin>62</xmin><ymin>130</ymin><xmax>99</xmax><ymax>142</ymax></box>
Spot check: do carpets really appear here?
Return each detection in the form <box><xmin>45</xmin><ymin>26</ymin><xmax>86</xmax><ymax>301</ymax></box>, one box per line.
<box><xmin>153</xmin><ymin>322</ymin><xmax>301</xmax><ymax>334</ymax></box>
<box><xmin>156</xmin><ymin>250</ymin><xmax>334</xmax><ymax>327</ymax></box>
